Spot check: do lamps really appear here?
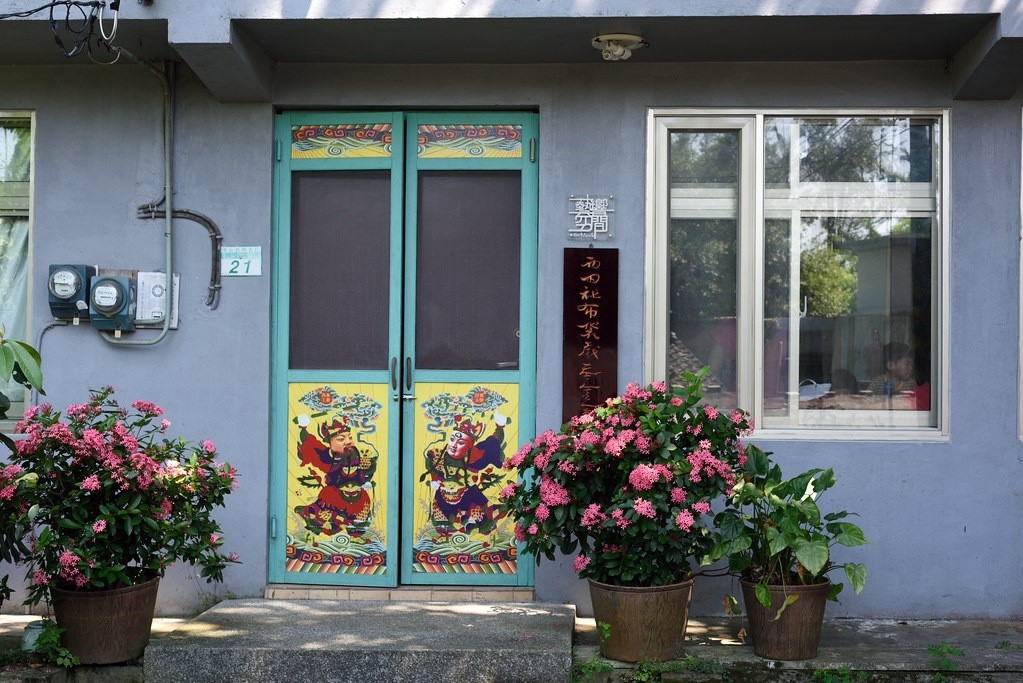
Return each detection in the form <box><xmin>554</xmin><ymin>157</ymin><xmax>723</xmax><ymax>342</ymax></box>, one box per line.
<box><xmin>592</xmin><ymin>34</ymin><xmax>644</xmax><ymax>63</ymax></box>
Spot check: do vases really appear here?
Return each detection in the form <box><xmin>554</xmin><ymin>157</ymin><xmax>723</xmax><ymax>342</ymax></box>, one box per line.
<box><xmin>47</xmin><ymin>566</ymin><xmax>162</xmax><ymax>665</ymax></box>
<box><xmin>742</xmin><ymin>575</ymin><xmax>831</xmax><ymax>659</ymax></box>
<box><xmin>591</xmin><ymin>571</ymin><xmax>696</xmax><ymax>663</ymax></box>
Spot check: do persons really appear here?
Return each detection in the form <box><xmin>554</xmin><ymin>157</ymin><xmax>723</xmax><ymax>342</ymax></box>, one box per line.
<box><xmin>867</xmin><ymin>342</ymin><xmax>919</xmax><ymax>395</ymax></box>
<box><xmin>708</xmin><ymin>274</ymin><xmax>788</xmax><ymax>410</ymax></box>
<box><xmin>821</xmin><ymin>369</ymin><xmax>858</xmax><ymax>395</ymax></box>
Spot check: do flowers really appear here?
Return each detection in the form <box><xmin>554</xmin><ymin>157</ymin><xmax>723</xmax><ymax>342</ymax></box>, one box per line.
<box><xmin>497</xmin><ymin>379</ymin><xmax>758</xmax><ymax>584</ymax></box>
<box><xmin>704</xmin><ymin>444</ymin><xmax>871</xmax><ymax>595</ymax></box>
<box><xmin>1</xmin><ymin>385</ymin><xmax>247</xmax><ymax>604</ymax></box>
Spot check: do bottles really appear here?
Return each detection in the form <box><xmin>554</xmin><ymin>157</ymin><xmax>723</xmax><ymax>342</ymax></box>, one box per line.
<box><xmin>884</xmin><ymin>380</ymin><xmax>894</xmax><ymax>397</ymax></box>
